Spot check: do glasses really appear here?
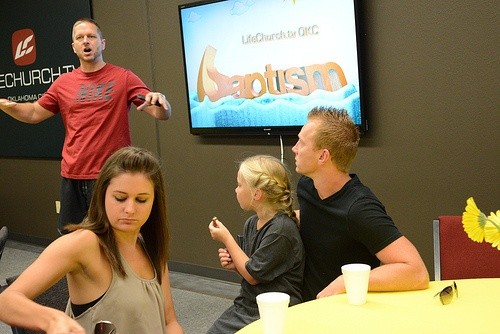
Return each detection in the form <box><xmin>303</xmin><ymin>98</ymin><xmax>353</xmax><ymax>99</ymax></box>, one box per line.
<box><xmin>94</xmin><ymin>320</ymin><xmax>116</xmax><ymax>334</ymax></box>
<box><xmin>434</xmin><ymin>281</ymin><xmax>458</xmax><ymax>305</ymax></box>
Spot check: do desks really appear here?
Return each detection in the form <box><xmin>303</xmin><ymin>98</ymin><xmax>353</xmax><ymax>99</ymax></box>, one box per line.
<box><xmin>232</xmin><ymin>278</ymin><xmax>500</xmax><ymax>334</ymax></box>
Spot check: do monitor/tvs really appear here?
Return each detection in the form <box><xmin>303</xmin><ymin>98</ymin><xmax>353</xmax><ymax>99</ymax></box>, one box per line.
<box><xmin>178</xmin><ymin>0</ymin><xmax>368</xmax><ymax>139</ymax></box>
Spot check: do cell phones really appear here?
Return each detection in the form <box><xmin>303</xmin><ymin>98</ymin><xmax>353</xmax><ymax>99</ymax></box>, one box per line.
<box><xmin>137</xmin><ymin>94</ymin><xmax>161</xmax><ymax>107</ymax></box>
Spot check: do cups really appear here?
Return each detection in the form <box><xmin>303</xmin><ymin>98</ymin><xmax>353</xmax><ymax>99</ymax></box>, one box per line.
<box><xmin>256</xmin><ymin>292</ymin><xmax>290</xmax><ymax>334</ymax></box>
<box><xmin>341</xmin><ymin>263</ymin><xmax>371</xmax><ymax>305</ymax></box>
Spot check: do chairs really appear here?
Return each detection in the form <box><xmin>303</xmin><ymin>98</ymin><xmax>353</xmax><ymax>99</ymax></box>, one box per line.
<box><xmin>433</xmin><ymin>215</ymin><xmax>500</xmax><ymax>280</ymax></box>
<box><xmin>0</xmin><ymin>226</ymin><xmax>8</xmax><ymax>261</ymax></box>
<box><xmin>0</xmin><ymin>264</ymin><xmax>69</xmax><ymax>334</ymax></box>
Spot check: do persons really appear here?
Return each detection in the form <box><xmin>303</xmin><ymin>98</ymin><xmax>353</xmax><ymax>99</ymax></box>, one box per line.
<box><xmin>206</xmin><ymin>155</ymin><xmax>305</xmax><ymax>334</ymax></box>
<box><xmin>0</xmin><ymin>18</ymin><xmax>171</xmax><ymax>236</ymax></box>
<box><xmin>0</xmin><ymin>146</ymin><xmax>184</xmax><ymax>333</ymax></box>
<box><xmin>293</xmin><ymin>106</ymin><xmax>429</xmax><ymax>302</ymax></box>
